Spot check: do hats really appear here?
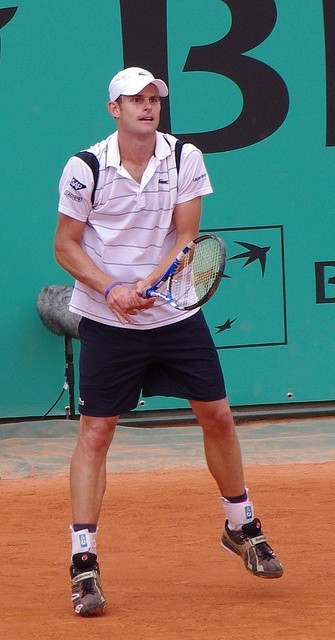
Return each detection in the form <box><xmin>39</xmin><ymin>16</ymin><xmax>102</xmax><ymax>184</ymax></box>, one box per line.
<box><xmin>109</xmin><ymin>67</ymin><xmax>169</xmax><ymax>102</ymax></box>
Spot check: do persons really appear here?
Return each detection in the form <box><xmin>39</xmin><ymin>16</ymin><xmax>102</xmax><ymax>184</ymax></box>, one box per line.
<box><xmin>54</xmin><ymin>68</ymin><xmax>283</xmax><ymax>618</ymax></box>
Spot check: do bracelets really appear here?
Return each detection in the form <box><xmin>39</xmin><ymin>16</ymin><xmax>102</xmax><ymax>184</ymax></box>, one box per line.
<box><xmin>105</xmin><ymin>282</ymin><xmax>123</xmax><ymax>300</ymax></box>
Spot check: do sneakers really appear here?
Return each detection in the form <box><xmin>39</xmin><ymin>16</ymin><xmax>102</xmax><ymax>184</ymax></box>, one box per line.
<box><xmin>221</xmin><ymin>517</ymin><xmax>284</xmax><ymax>578</ymax></box>
<box><xmin>69</xmin><ymin>553</ymin><xmax>106</xmax><ymax>615</ymax></box>
